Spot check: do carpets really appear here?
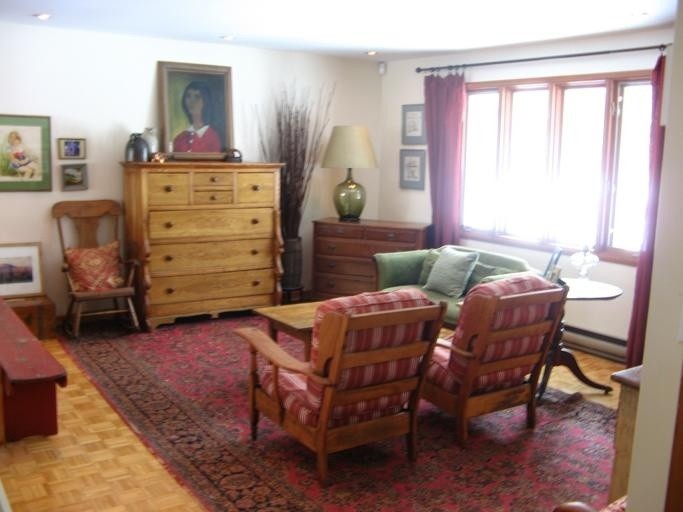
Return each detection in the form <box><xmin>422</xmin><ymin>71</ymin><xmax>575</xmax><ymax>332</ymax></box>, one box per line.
<box><xmin>47</xmin><ymin>306</ymin><xmax>618</xmax><ymax>512</ymax></box>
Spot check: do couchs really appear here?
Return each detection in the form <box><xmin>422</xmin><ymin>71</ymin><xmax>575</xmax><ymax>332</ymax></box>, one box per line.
<box><xmin>367</xmin><ymin>246</ymin><xmax>546</xmax><ymax>330</ymax></box>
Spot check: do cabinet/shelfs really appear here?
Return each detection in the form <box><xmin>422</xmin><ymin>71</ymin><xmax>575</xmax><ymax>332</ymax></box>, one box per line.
<box><xmin>310</xmin><ymin>217</ymin><xmax>434</xmax><ymax>302</ymax></box>
<box><xmin>117</xmin><ymin>158</ymin><xmax>286</xmax><ymax>334</ymax></box>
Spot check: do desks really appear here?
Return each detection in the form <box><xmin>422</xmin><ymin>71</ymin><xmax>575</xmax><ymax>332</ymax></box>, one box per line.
<box><xmin>5</xmin><ymin>297</ymin><xmax>56</xmax><ymax>342</ymax></box>
<box><xmin>534</xmin><ymin>272</ymin><xmax>625</xmax><ymax>407</ymax></box>
<box><xmin>602</xmin><ymin>361</ymin><xmax>646</xmax><ymax>507</ymax></box>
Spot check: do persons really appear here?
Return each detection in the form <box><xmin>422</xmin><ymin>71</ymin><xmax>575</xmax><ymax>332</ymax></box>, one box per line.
<box><xmin>171</xmin><ymin>81</ymin><xmax>223</xmax><ymax>152</ymax></box>
<box><xmin>3</xmin><ymin>131</ymin><xmax>41</xmax><ymax>179</ymax></box>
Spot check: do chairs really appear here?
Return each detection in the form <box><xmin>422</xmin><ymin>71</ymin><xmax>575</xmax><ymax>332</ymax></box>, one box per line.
<box><xmin>50</xmin><ymin>197</ymin><xmax>145</xmax><ymax>341</ymax></box>
<box><xmin>421</xmin><ymin>267</ymin><xmax>569</xmax><ymax>451</ymax></box>
<box><xmin>234</xmin><ymin>283</ymin><xmax>450</xmax><ymax>488</ymax></box>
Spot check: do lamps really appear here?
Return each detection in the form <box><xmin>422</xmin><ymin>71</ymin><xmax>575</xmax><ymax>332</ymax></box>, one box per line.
<box><xmin>320</xmin><ymin>124</ymin><xmax>381</xmax><ymax>222</ymax></box>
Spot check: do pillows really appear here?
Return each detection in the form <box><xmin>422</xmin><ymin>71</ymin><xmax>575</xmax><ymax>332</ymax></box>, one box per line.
<box><xmin>61</xmin><ymin>238</ymin><xmax>127</xmax><ymax>295</ymax></box>
<box><xmin>423</xmin><ymin>243</ymin><xmax>482</xmax><ymax>301</ymax></box>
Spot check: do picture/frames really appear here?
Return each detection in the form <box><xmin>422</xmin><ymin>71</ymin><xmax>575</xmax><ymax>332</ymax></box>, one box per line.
<box><xmin>0</xmin><ymin>239</ymin><xmax>47</xmax><ymax>298</ymax></box>
<box><xmin>398</xmin><ymin>102</ymin><xmax>426</xmax><ymax>147</ymax></box>
<box><xmin>59</xmin><ymin>163</ymin><xmax>89</xmax><ymax>193</ymax></box>
<box><xmin>398</xmin><ymin>147</ymin><xmax>427</xmax><ymax>193</ymax></box>
<box><xmin>155</xmin><ymin>58</ymin><xmax>234</xmax><ymax>163</ymax></box>
<box><xmin>56</xmin><ymin>136</ymin><xmax>88</xmax><ymax>161</ymax></box>
<box><xmin>0</xmin><ymin>113</ymin><xmax>52</xmax><ymax>193</ymax></box>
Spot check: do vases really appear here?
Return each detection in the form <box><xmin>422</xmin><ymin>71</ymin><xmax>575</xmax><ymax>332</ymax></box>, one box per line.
<box><xmin>279</xmin><ymin>234</ymin><xmax>304</xmax><ymax>291</ymax></box>
<box><xmin>138</xmin><ymin>127</ymin><xmax>157</xmax><ymax>158</ymax></box>
<box><xmin>122</xmin><ymin>132</ymin><xmax>150</xmax><ymax>163</ymax></box>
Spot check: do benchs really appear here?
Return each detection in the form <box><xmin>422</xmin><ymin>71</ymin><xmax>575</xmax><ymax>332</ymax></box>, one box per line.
<box><xmin>0</xmin><ymin>294</ymin><xmax>68</xmax><ymax>448</ymax></box>
